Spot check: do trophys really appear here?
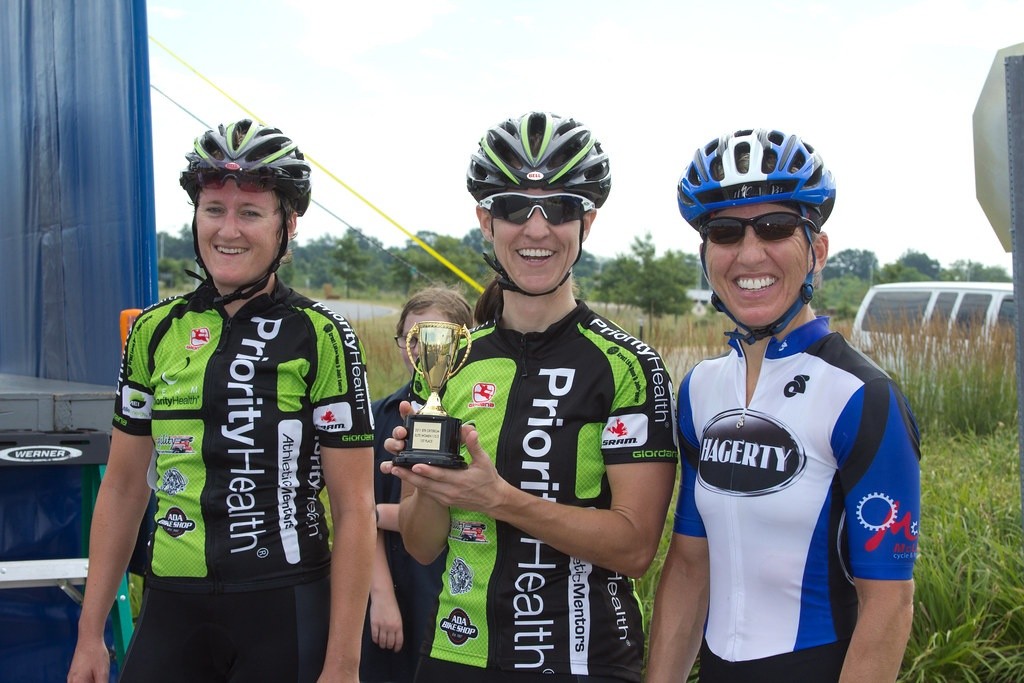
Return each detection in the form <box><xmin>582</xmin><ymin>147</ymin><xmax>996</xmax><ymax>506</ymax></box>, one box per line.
<box><xmin>393</xmin><ymin>319</ymin><xmax>473</xmax><ymax>470</ymax></box>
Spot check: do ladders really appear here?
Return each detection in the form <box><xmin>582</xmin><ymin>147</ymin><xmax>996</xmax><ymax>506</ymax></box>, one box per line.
<box><xmin>0</xmin><ymin>430</ymin><xmax>134</xmax><ymax>683</ymax></box>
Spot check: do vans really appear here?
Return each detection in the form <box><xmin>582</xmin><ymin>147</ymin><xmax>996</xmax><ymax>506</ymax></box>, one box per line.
<box><xmin>848</xmin><ymin>278</ymin><xmax>1018</xmax><ymax>402</ymax></box>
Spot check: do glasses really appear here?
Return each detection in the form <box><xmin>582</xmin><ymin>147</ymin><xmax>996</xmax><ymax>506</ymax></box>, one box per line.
<box><xmin>478</xmin><ymin>192</ymin><xmax>596</xmax><ymax>225</ymax></box>
<box><xmin>698</xmin><ymin>210</ymin><xmax>818</xmax><ymax>245</ymax></box>
<box><xmin>191</xmin><ymin>163</ymin><xmax>295</xmax><ymax>193</ymax></box>
<box><xmin>394</xmin><ymin>335</ymin><xmax>418</xmax><ymax>350</ymax></box>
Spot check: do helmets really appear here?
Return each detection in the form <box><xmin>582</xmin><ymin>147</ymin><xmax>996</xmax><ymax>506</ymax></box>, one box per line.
<box><xmin>677</xmin><ymin>129</ymin><xmax>835</xmax><ymax>231</ymax></box>
<box><xmin>179</xmin><ymin>116</ymin><xmax>311</xmax><ymax>218</ymax></box>
<box><xmin>467</xmin><ymin>109</ymin><xmax>611</xmax><ymax>211</ymax></box>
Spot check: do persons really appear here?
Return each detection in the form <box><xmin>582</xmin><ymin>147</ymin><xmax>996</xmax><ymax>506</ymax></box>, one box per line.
<box><xmin>358</xmin><ymin>287</ymin><xmax>480</xmax><ymax>683</ymax></box>
<box><xmin>66</xmin><ymin>117</ymin><xmax>378</xmax><ymax>682</ymax></box>
<box><xmin>399</xmin><ymin>109</ymin><xmax>680</xmax><ymax>683</ymax></box>
<box><xmin>646</xmin><ymin>127</ymin><xmax>923</xmax><ymax>683</ymax></box>
